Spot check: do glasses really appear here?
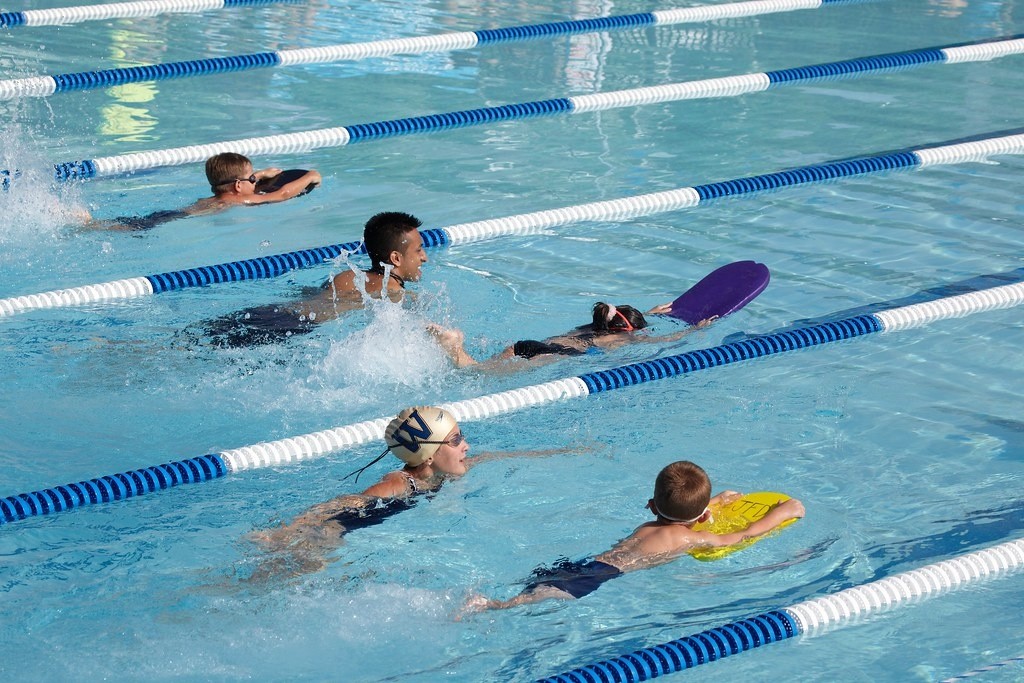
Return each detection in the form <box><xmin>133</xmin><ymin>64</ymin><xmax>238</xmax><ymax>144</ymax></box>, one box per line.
<box><xmin>235</xmin><ymin>173</ymin><xmax>256</xmax><ymax>184</ymax></box>
<box><xmin>442</xmin><ymin>430</ymin><xmax>464</xmax><ymax>448</ymax></box>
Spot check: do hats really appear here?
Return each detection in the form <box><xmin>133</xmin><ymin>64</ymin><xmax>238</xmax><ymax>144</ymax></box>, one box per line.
<box><xmin>385</xmin><ymin>407</ymin><xmax>457</xmax><ymax>469</ymax></box>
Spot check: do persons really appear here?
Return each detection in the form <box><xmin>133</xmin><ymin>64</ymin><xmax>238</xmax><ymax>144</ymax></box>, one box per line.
<box><xmin>185</xmin><ymin>211</ymin><xmax>429</xmax><ymax>356</ymax></box>
<box><xmin>424</xmin><ymin>301</ymin><xmax>720</xmax><ymax>386</ymax></box>
<box><xmin>454</xmin><ymin>460</ymin><xmax>805</xmax><ymax>624</ymax></box>
<box><xmin>74</xmin><ymin>153</ymin><xmax>323</xmax><ymax>232</ymax></box>
<box><xmin>192</xmin><ymin>405</ymin><xmax>607</xmax><ymax>599</ymax></box>
